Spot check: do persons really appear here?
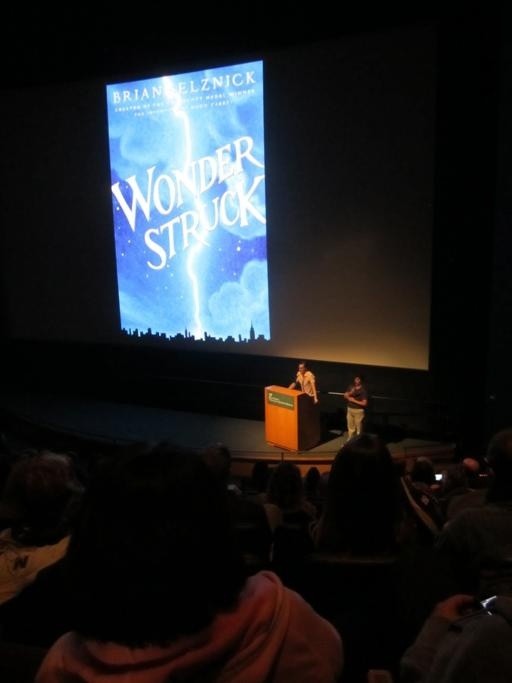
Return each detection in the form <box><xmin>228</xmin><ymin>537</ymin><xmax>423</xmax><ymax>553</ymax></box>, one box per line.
<box><xmin>344</xmin><ymin>376</ymin><xmax>368</xmax><ymax>441</ymax></box>
<box><xmin>288</xmin><ymin>361</ymin><xmax>320</xmax><ymax>404</ymax></box>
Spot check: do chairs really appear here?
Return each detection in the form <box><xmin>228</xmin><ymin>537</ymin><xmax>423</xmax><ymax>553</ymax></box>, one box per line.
<box><xmin>236</xmin><ymin>490</ymin><xmax>445</xmax><ymax>681</ymax></box>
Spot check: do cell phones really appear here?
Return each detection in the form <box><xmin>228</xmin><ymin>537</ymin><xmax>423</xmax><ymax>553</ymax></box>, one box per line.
<box><xmin>481</xmin><ymin>595</ymin><xmax>498</xmax><ymax>617</ymax></box>
<box><xmin>434</xmin><ymin>473</ymin><xmax>446</xmax><ymax>481</ymax></box>
